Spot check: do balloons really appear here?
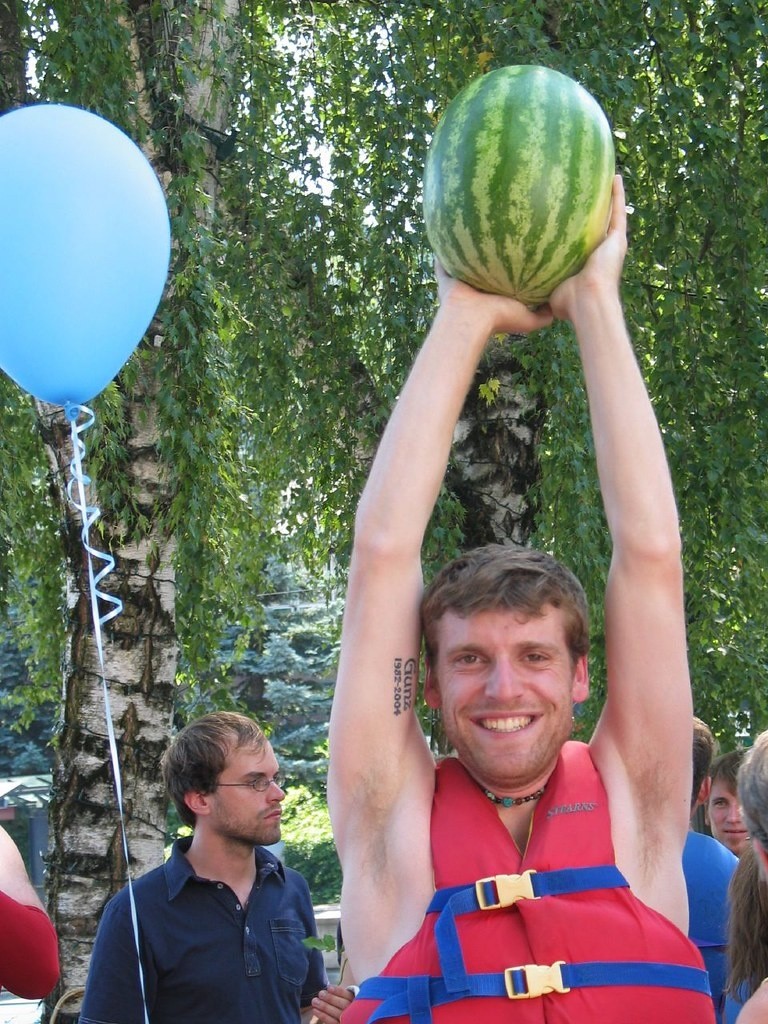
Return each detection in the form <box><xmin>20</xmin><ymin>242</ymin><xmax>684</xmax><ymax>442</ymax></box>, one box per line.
<box><xmin>0</xmin><ymin>101</ymin><xmax>172</xmax><ymax>406</ymax></box>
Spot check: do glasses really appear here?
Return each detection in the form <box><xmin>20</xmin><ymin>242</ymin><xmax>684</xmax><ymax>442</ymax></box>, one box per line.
<box><xmin>204</xmin><ymin>773</ymin><xmax>285</xmax><ymax>792</ymax></box>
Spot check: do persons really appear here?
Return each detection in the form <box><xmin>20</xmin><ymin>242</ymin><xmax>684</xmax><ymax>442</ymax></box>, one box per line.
<box><xmin>682</xmin><ymin>713</ymin><xmax>740</xmax><ymax>1022</ymax></box>
<box><xmin>0</xmin><ymin>824</ymin><xmax>61</xmax><ymax>998</ymax></box>
<box><xmin>728</xmin><ymin>730</ymin><xmax>768</xmax><ymax>1024</ymax></box>
<box><xmin>320</xmin><ymin>170</ymin><xmax>719</xmax><ymax>1024</ymax></box>
<box><xmin>76</xmin><ymin>708</ymin><xmax>356</xmax><ymax>1024</ymax></box>
<box><xmin>703</xmin><ymin>746</ymin><xmax>758</xmax><ymax>856</ymax></box>
<box><xmin>723</xmin><ymin>839</ymin><xmax>768</xmax><ymax>1024</ymax></box>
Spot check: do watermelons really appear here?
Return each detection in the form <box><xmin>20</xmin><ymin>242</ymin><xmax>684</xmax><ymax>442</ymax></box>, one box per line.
<box><xmin>422</xmin><ymin>65</ymin><xmax>614</xmax><ymax>304</ymax></box>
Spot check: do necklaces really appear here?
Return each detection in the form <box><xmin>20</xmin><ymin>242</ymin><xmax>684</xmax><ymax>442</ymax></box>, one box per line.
<box><xmin>474</xmin><ymin>782</ymin><xmax>548</xmax><ymax>807</ymax></box>
